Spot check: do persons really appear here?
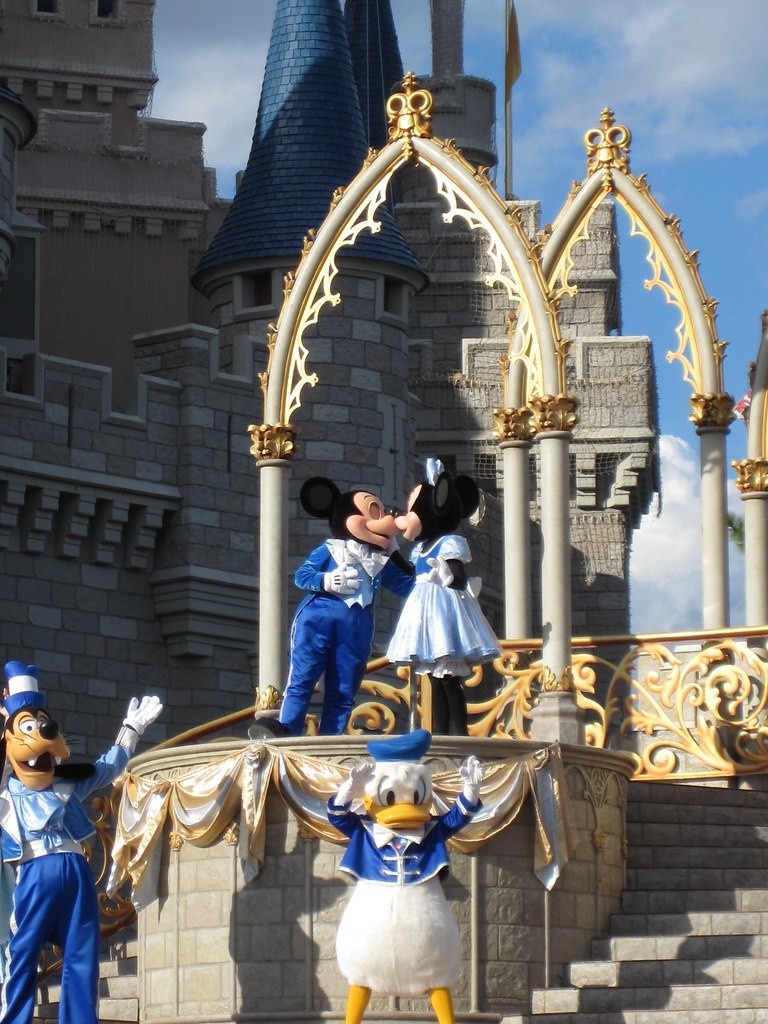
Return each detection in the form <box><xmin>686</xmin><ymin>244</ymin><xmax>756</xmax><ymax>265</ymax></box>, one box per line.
<box><xmin>248</xmin><ymin>477</ymin><xmax>482</xmax><ymax>739</ymax></box>
<box><xmin>0</xmin><ymin>661</ymin><xmax>163</xmax><ymax>1024</ymax></box>
<box><xmin>326</xmin><ymin>729</ymin><xmax>485</xmax><ymax>1024</ymax></box>
<box><xmin>387</xmin><ymin>458</ymin><xmax>505</xmax><ymax>736</ymax></box>
